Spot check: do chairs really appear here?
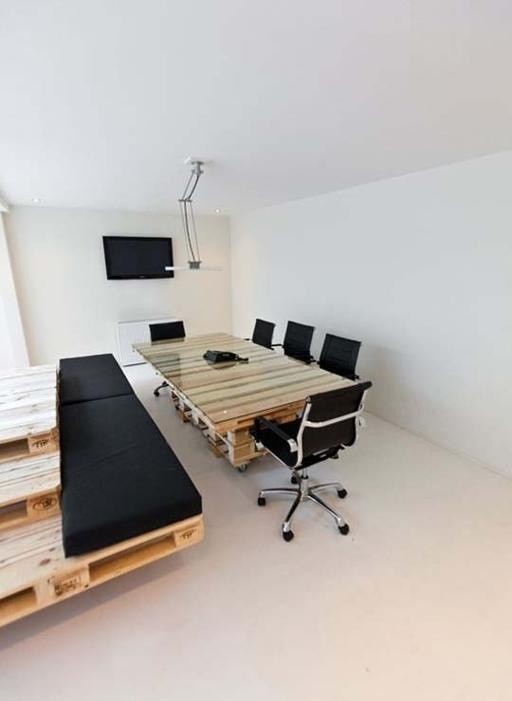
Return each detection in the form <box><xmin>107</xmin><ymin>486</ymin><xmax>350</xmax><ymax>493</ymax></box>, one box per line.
<box><xmin>248</xmin><ymin>382</ymin><xmax>366</xmax><ymax>543</ymax></box>
<box><xmin>242</xmin><ymin>317</ymin><xmax>363</xmax><ymax>382</ymax></box>
<box><xmin>148</xmin><ymin>321</ymin><xmax>186</xmax><ymax>398</ymax></box>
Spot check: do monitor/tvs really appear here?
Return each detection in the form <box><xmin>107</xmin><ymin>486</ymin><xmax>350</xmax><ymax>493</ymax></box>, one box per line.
<box><xmin>102</xmin><ymin>235</ymin><xmax>174</xmax><ymax>280</ymax></box>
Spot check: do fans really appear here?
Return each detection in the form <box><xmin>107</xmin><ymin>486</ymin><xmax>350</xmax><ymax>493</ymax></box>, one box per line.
<box><xmin>165</xmin><ymin>158</ymin><xmax>225</xmax><ymax>273</ymax></box>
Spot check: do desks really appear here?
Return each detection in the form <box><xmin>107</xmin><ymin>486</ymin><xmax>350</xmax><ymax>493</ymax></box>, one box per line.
<box><xmin>131</xmin><ymin>331</ymin><xmax>358</xmax><ymax>473</ymax></box>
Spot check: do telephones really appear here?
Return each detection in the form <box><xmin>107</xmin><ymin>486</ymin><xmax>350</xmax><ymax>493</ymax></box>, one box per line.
<box><xmin>203</xmin><ymin>349</ymin><xmax>240</xmax><ymax>362</ymax></box>
